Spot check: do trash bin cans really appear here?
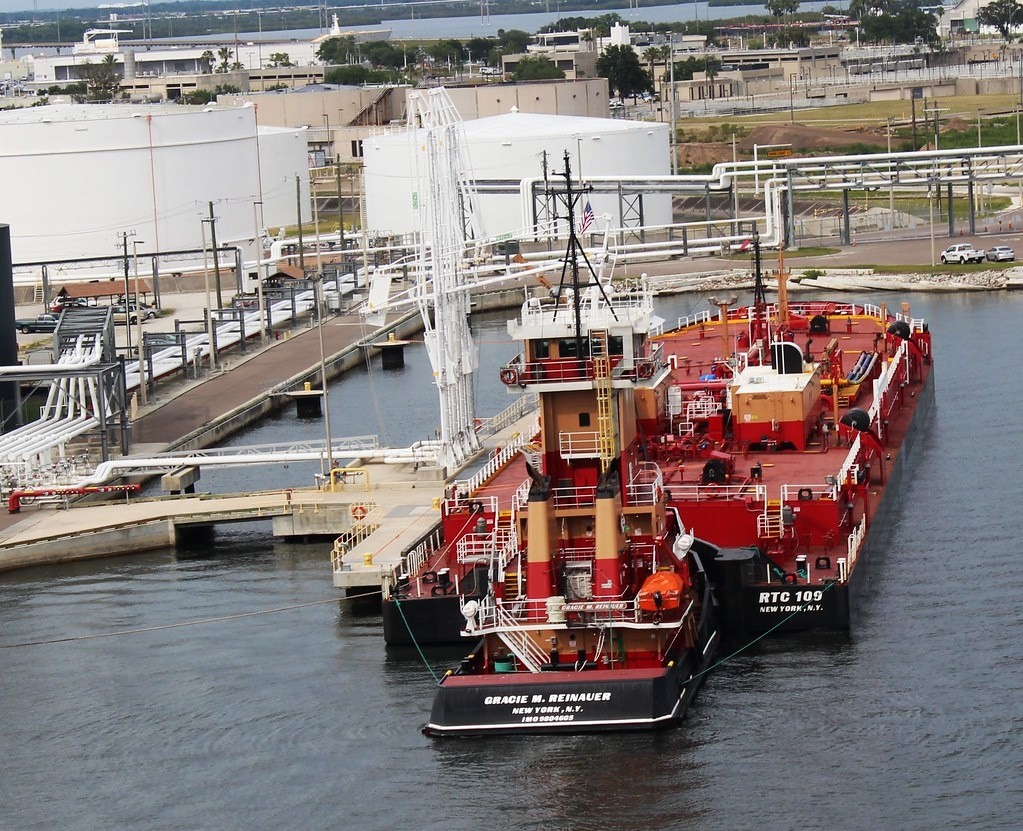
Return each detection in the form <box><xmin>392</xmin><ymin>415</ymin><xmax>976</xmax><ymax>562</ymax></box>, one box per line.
<box><xmin>490</xmin><ymin>238</ymin><xmax>520</xmax><ymax>271</ymax></box>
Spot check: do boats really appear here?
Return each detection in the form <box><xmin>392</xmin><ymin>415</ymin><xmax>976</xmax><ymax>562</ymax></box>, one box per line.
<box><xmin>637</xmin><ymin>571</ymin><xmax>687</xmax><ymax>612</ymax></box>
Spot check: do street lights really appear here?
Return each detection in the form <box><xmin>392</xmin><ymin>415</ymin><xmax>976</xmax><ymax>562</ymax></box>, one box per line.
<box><xmin>1007</xmin><ymin>65</ymin><xmax>1016</xmax><ymax>114</ymax></box>
<box><xmin>731</xmin><ymin>131</ymin><xmax>741</xmax><ymax>245</ymax></box>
<box><xmin>665</xmin><ymin>29</ymin><xmax>680</xmax><ymax>175</ymax></box>
<box><xmin>200</xmin><ymin>218</ymin><xmax>216</xmax><ymax>371</ymax></box>
<box><xmin>253</xmin><ymin>201</ymin><xmax>266</xmax><ymax>345</ymax></box>
<box><xmin>790</xmin><ymin>72</ymin><xmax>797</xmax><ymax>123</ymax></box>
<box><xmin>312</xmin><ymin>182</ymin><xmax>323</xmax><ymax>316</ymax></box>
<box><xmin>1016</xmin><ymin>102</ymin><xmax>1023</xmax><ymax>155</ymax></box>
<box><xmin>978</xmin><ymin>108</ymin><xmax>987</xmax><ymax>173</ymax></box>
<box><xmin>576</xmin><ymin>138</ymin><xmax>587</xmax><ymax>248</ymax></box>
<box><xmin>132</xmin><ymin>240</ymin><xmax>148</xmax><ymax>406</ymax></box>
<box><xmin>888</xmin><ymin>117</ymin><xmax>894</xmax><ymax>179</ymax></box>
<box><xmin>322</xmin><ymin>114</ymin><xmax>332</xmax><ymax>166</ymax></box>
<box><xmin>303</xmin><ymin>272</ymin><xmax>337</xmax><ymax>484</ymax></box>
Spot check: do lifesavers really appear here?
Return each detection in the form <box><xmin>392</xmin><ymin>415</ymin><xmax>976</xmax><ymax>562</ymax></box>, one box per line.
<box><xmin>501</xmin><ymin>369</ymin><xmax>516</xmax><ymax>385</ymax></box>
<box><xmin>473</xmin><ymin>418</ymin><xmax>482</xmax><ymax>431</ymax></box>
<box><xmin>826</xmin><ymin>303</ymin><xmax>836</xmax><ymax>314</ymax></box>
<box><xmin>737</xmin><ymin>306</ymin><xmax>748</xmax><ymax>317</ymax></box>
<box><xmin>352</xmin><ymin>506</ymin><xmax>366</xmax><ymax>519</ymax></box>
<box><xmin>638</xmin><ymin>362</ymin><xmax>654</xmax><ymax>378</ymax></box>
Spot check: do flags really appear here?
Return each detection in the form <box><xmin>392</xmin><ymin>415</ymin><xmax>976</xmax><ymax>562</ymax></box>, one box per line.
<box><xmin>739</xmin><ymin>239</ymin><xmax>750</xmax><ymax>252</ymax></box>
<box><xmin>577</xmin><ymin>201</ymin><xmax>595</xmax><ymax>235</ymax></box>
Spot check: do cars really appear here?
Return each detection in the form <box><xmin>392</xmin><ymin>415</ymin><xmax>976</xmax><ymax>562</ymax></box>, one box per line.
<box><xmin>985</xmin><ymin>245</ymin><xmax>1015</xmax><ymax>262</ymax></box>
<box><xmin>46</xmin><ymin>295</ymin><xmax>162</xmax><ymax>325</ymax></box>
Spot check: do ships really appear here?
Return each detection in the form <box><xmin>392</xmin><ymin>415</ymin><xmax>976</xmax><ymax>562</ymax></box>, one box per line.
<box><xmin>378</xmin><ymin>147</ymin><xmax>933</xmax><ymax>741</ymax></box>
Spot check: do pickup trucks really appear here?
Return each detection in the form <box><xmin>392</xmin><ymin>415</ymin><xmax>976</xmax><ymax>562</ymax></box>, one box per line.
<box><xmin>16</xmin><ymin>314</ymin><xmax>61</xmax><ymax>335</ymax></box>
<box><xmin>940</xmin><ymin>243</ymin><xmax>986</xmax><ymax>264</ymax></box>
